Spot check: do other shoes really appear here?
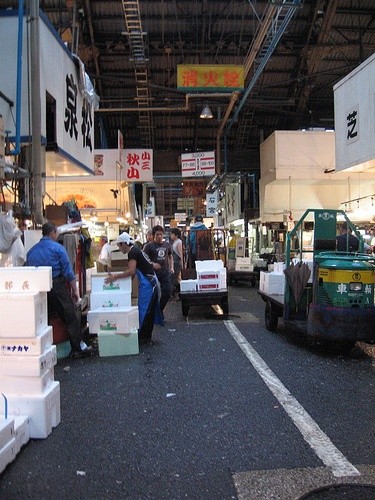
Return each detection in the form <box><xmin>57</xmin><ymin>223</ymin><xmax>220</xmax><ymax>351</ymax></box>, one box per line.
<box><xmin>158</xmin><ymin>310</ymin><xmax>166</xmax><ymax>320</ymax></box>
<box><xmin>139</xmin><ymin>337</ymin><xmax>151</xmax><ymax>342</ymax></box>
<box><xmin>72</xmin><ymin>352</ymin><xmax>87</xmax><ymax>359</ymax></box>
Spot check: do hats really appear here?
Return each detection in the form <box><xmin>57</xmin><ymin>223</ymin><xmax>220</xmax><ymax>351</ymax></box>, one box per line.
<box><xmin>112</xmin><ymin>232</ymin><xmax>135</xmax><ymax>245</ymax></box>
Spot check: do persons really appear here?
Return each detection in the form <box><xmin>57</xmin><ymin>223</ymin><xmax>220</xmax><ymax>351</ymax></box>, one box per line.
<box><xmin>186</xmin><ymin>215</ymin><xmax>213</xmax><ymax>269</ymax></box>
<box><xmin>26</xmin><ymin>222</ymin><xmax>90</xmax><ymax>358</ymax></box>
<box><xmin>143</xmin><ymin>231</ymin><xmax>152</xmax><ymax>246</ymax></box>
<box><xmin>104</xmin><ymin>233</ymin><xmax>158</xmax><ymax>346</ymax></box>
<box><xmin>336</xmin><ymin>222</ymin><xmax>359</xmax><ymax>256</ymax></box>
<box><xmin>143</xmin><ymin>225</ymin><xmax>175</xmax><ymax>320</ymax></box>
<box><xmin>170</xmin><ymin>228</ymin><xmax>186</xmax><ymax>300</ymax></box>
<box><xmin>355</xmin><ymin>228</ymin><xmax>375</xmax><ymax>256</ymax></box>
<box><xmin>229</xmin><ymin>229</ymin><xmax>236</xmax><ymax>247</ymax></box>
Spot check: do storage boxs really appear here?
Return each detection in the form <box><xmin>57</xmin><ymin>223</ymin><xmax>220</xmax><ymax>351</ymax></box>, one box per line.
<box><xmin>0</xmin><ymin>266</ymin><xmax>61</xmax><ymax>473</ymax></box>
<box><xmin>260</xmin><ymin>258</ymin><xmax>313</xmax><ymax>295</ymax></box>
<box><xmin>180</xmin><ymin>260</ymin><xmax>227</xmax><ymax>292</ymax></box>
<box><xmin>235</xmin><ymin>257</ymin><xmax>253</xmax><ymax>271</ymax></box>
<box><xmin>87</xmin><ymin>249</ymin><xmax>139</xmax><ymax>357</ymax></box>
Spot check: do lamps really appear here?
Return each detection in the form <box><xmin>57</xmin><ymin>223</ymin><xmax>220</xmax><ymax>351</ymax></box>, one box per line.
<box><xmin>200</xmin><ymin>107</ymin><xmax>213</xmax><ymax>118</ymax></box>
